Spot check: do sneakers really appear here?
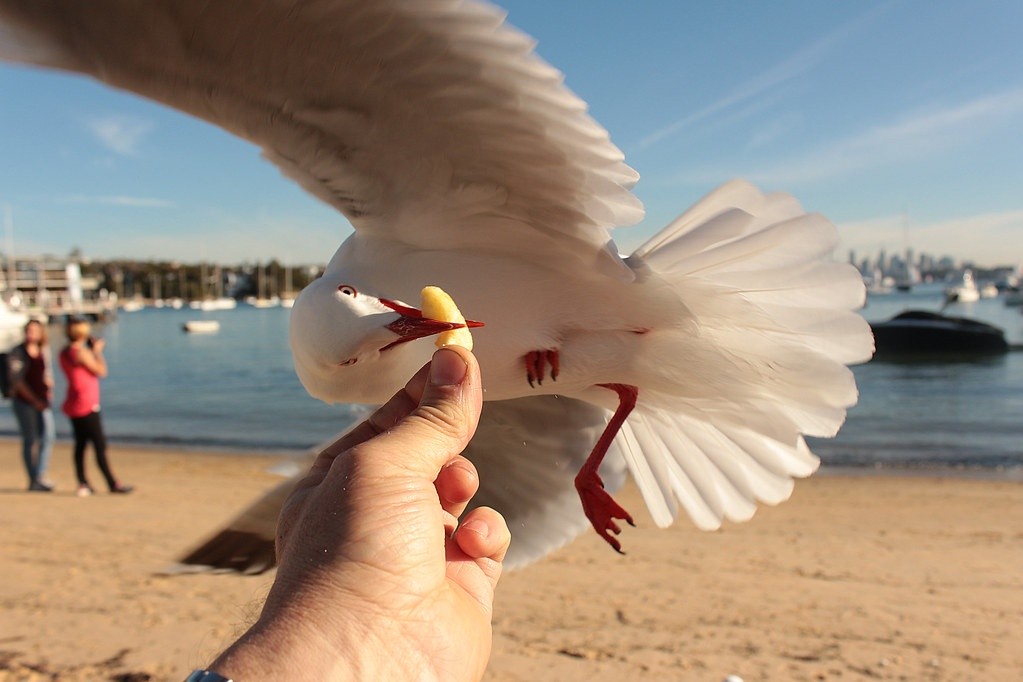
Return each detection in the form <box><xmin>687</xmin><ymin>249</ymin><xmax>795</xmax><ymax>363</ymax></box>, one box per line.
<box><xmin>74</xmin><ymin>482</ymin><xmax>98</xmax><ymax>495</ymax></box>
<box><xmin>107</xmin><ymin>480</ymin><xmax>135</xmax><ymax>493</ymax></box>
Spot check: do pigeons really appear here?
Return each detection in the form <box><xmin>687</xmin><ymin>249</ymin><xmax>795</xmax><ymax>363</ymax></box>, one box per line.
<box><xmin>3</xmin><ymin>0</ymin><xmax>877</xmax><ymax>578</ymax></box>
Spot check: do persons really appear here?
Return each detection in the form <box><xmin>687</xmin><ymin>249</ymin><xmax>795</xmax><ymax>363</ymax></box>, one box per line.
<box><xmin>176</xmin><ymin>353</ymin><xmax>513</xmax><ymax>682</ymax></box>
<box><xmin>7</xmin><ymin>320</ymin><xmax>55</xmax><ymax>493</ymax></box>
<box><xmin>59</xmin><ymin>313</ymin><xmax>135</xmax><ymax>496</ymax></box>
